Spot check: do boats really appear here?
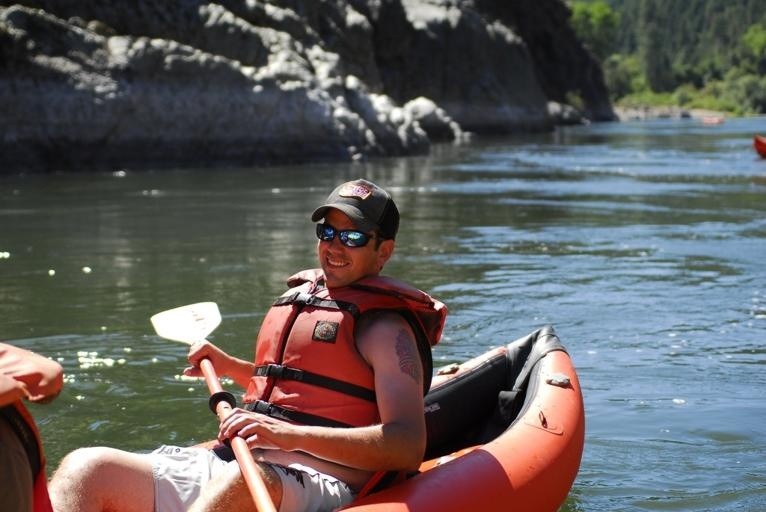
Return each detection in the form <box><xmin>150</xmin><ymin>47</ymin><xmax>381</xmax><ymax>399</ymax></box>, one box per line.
<box><xmin>332</xmin><ymin>324</ymin><xmax>588</xmax><ymax>512</ymax></box>
<box><xmin>755</xmin><ymin>134</ymin><xmax>766</xmax><ymax>158</ymax></box>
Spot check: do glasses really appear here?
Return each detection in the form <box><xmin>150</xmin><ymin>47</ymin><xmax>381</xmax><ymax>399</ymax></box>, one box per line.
<box><xmin>316</xmin><ymin>223</ymin><xmax>384</xmax><ymax>248</ymax></box>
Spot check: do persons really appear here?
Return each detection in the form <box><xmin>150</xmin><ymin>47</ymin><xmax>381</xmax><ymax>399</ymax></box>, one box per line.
<box><xmin>47</xmin><ymin>176</ymin><xmax>447</xmax><ymax>511</ymax></box>
<box><xmin>0</xmin><ymin>339</ymin><xmax>66</xmax><ymax>512</ymax></box>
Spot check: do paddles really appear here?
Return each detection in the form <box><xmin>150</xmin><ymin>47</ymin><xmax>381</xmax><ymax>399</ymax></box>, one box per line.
<box><xmin>151</xmin><ymin>301</ymin><xmax>277</xmax><ymax>512</ymax></box>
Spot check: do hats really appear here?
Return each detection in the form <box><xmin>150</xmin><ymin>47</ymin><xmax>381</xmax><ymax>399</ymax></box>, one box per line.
<box><xmin>312</xmin><ymin>179</ymin><xmax>399</xmax><ymax>240</ymax></box>
